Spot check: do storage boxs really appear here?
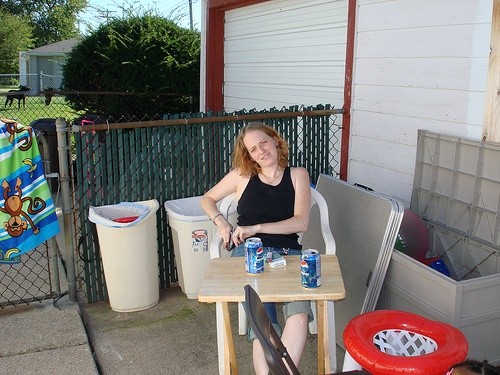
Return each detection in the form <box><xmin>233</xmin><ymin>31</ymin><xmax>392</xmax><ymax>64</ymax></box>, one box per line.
<box><xmin>374</xmin><ymin>129</ymin><xmax>500</xmax><ymax>367</ymax></box>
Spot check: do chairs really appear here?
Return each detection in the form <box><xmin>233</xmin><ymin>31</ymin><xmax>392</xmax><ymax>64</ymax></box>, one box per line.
<box><xmin>209</xmin><ymin>187</ymin><xmax>337</xmax><ymax>375</ymax></box>
<box><xmin>243</xmin><ymin>285</ymin><xmax>299</xmax><ymax>375</ymax></box>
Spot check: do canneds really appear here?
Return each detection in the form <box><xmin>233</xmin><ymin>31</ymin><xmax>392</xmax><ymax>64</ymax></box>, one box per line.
<box><xmin>244</xmin><ymin>237</ymin><xmax>265</xmax><ymax>274</ymax></box>
<box><xmin>300</xmin><ymin>248</ymin><xmax>322</xmax><ymax>289</ymax></box>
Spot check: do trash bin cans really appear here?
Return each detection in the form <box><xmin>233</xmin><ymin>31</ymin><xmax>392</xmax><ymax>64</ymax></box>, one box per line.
<box><xmin>88</xmin><ymin>199</ymin><xmax>160</xmax><ymax>313</ymax></box>
<box><xmin>164</xmin><ymin>195</ymin><xmax>239</xmax><ymax>300</ymax></box>
<box><xmin>27</xmin><ymin>117</ymin><xmax>68</xmax><ymax>193</ymax></box>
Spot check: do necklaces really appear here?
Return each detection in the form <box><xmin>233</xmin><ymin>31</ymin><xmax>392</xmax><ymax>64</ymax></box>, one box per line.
<box><xmin>260</xmin><ymin>168</ymin><xmax>283</xmax><ymax>184</ymax></box>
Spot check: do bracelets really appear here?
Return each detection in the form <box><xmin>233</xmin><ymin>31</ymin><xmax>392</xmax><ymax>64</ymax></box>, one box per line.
<box><xmin>212</xmin><ymin>214</ymin><xmax>224</xmax><ymax>225</ymax></box>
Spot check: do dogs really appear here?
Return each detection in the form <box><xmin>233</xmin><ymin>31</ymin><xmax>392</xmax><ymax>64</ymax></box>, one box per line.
<box><xmin>4</xmin><ymin>85</ymin><xmax>32</xmax><ymax>109</ymax></box>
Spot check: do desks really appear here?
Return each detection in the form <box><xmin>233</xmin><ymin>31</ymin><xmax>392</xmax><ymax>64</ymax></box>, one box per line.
<box><xmin>198</xmin><ymin>254</ymin><xmax>346</xmax><ymax>375</ymax></box>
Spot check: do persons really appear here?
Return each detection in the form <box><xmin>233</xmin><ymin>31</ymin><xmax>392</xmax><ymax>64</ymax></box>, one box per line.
<box><xmin>200</xmin><ymin>123</ymin><xmax>316</xmax><ymax>375</ymax></box>
